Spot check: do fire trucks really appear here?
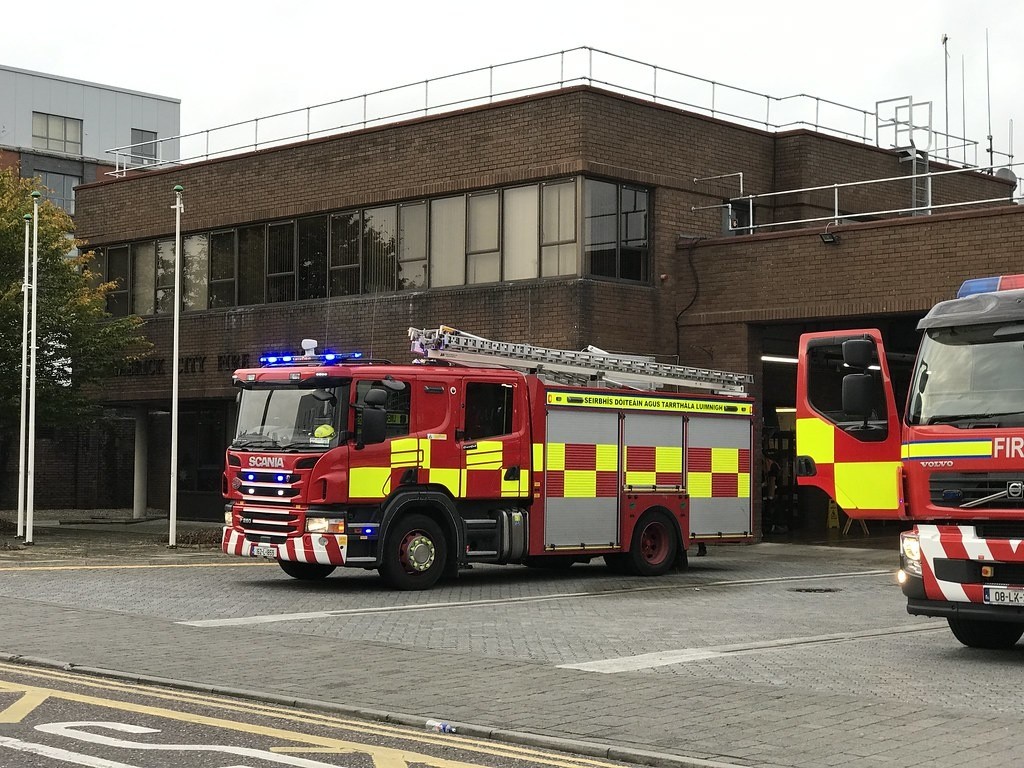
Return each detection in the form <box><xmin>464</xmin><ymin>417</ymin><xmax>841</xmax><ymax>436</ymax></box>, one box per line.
<box><xmin>794</xmin><ymin>268</ymin><xmax>1024</xmax><ymax>666</ymax></box>
<box><xmin>216</xmin><ymin>324</ymin><xmax>759</xmax><ymax>595</ymax></box>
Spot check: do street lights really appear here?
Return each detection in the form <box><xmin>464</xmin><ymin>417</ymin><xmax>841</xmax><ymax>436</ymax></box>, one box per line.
<box><xmin>39</xmin><ymin>535</ymin><xmax>43</xmax><ymax>541</ymax></box>
<box><xmin>16</xmin><ymin>189</ymin><xmax>43</xmax><ymax>545</ymax></box>
<box><xmin>169</xmin><ymin>183</ymin><xmax>185</xmax><ymax>550</ymax></box>
